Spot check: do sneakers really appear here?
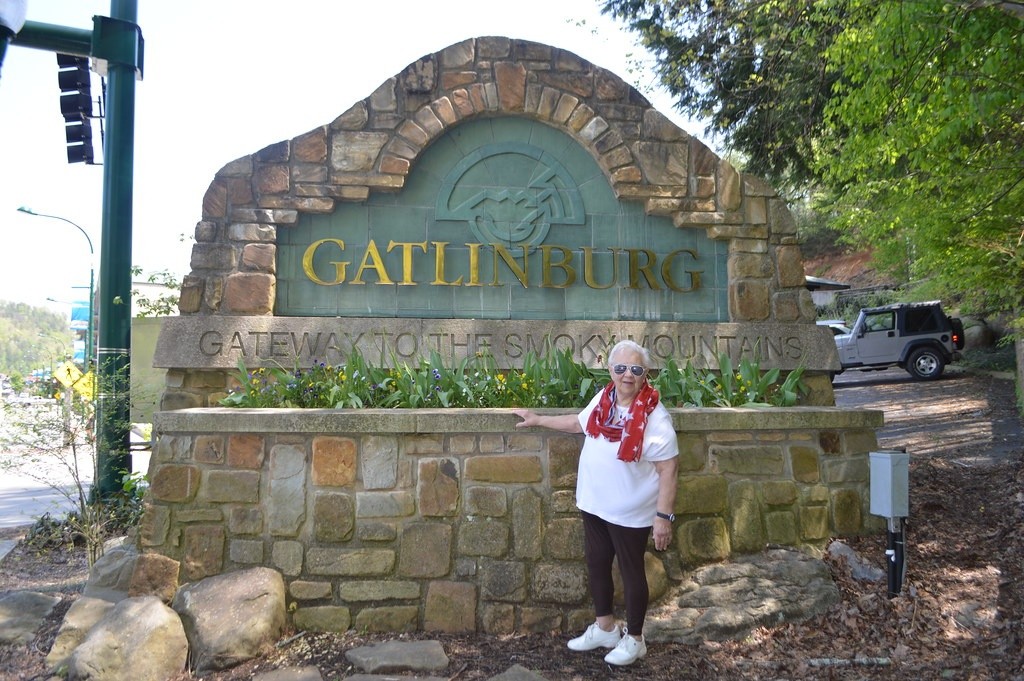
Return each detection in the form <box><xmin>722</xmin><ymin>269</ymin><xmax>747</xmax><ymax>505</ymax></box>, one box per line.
<box><xmin>567</xmin><ymin>622</ymin><xmax>620</xmax><ymax>650</ymax></box>
<box><xmin>604</xmin><ymin>627</ymin><xmax>647</xmax><ymax>665</ymax></box>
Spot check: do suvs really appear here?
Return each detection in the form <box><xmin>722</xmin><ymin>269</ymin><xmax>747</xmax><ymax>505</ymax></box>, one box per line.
<box><xmin>830</xmin><ymin>299</ymin><xmax>965</xmax><ymax>382</ymax></box>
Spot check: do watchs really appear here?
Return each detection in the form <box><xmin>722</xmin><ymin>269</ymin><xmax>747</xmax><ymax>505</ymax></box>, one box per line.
<box><xmin>656</xmin><ymin>511</ymin><xmax>675</xmax><ymax>523</ymax></box>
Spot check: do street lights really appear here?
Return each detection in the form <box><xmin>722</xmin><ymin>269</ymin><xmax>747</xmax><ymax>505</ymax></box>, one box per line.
<box><xmin>44</xmin><ymin>296</ymin><xmax>87</xmax><ymax>429</ymax></box>
<box><xmin>17</xmin><ymin>206</ymin><xmax>94</xmax><ymax>361</ymax></box>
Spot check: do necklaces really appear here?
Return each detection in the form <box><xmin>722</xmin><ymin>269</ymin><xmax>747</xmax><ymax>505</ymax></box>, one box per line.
<box><xmin>616</xmin><ymin>395</ymin><xmax>636</xmax><ymax>418</ymax></box>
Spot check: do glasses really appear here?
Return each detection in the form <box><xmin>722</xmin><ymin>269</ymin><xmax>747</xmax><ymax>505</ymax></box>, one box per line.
<box><xmin>610</xmin><ymin>364</ymin><xmax>646</xmax><ymax>377</ymax></box>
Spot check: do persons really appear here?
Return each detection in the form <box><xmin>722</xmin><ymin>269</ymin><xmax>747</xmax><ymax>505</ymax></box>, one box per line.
<box><xmin>512</xmin><ymin>339</ymin><xmax>680</xmax><ymax>667</ymax></box>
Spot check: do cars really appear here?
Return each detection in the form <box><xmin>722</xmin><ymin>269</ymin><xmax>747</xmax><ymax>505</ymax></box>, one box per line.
<box><xmin>815</xmin><ymin>320</ymin><xmax>851</xmax><ymax>339</ymax></box>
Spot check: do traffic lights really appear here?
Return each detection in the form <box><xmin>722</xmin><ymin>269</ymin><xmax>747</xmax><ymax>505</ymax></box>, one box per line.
<box><xmin>55</xmin><ymin>51</ymin><xmax>93</xmax><ymax>167</ymax></box>
